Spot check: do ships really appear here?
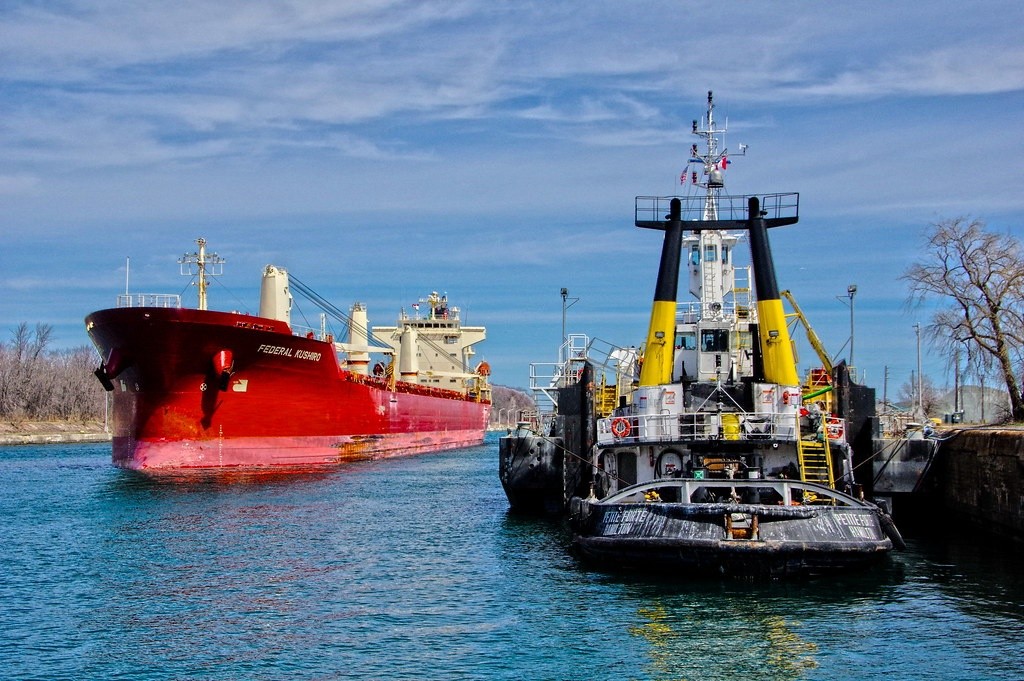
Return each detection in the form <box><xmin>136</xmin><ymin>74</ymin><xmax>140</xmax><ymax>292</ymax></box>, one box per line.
<box><xmin>498</xmin><ymin>90</ymin><xmax>941</xmax><ymax>577</ymax></box>
<box><xmin>84</xmin><ymin>235</ymin><xmax>512</xmax><ymax>476</ymax></box>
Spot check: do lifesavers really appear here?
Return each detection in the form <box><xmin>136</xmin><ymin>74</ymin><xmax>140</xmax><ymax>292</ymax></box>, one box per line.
<box><xmin>611</xmin><ymin>418</ymin><xmax>630</xmax><ymax>437</ymax></box>
<box><xmin>826</xmin><ymin>419</ymin><xmax>843</xmax><ymax>439</ymax></box>
